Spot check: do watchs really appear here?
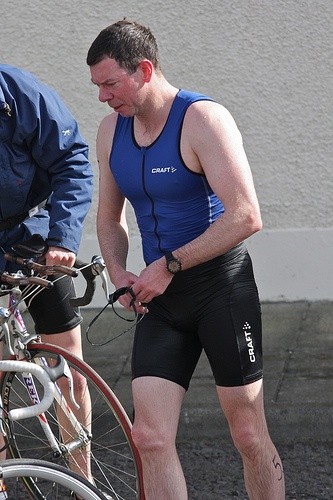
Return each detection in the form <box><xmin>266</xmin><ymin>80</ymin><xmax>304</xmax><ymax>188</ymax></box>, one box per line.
<box><xmin>165</xmin><ymin>251</ymin><xmax>182</xmax><ymax>276</ymax></box>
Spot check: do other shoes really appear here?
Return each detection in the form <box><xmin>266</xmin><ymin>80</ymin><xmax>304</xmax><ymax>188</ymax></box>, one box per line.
<box><xmin>0</xmin><ymin>485</ymin><xmax>8</xmax><ymax>500</ymax></box>
<box><xmin>73</xmin><ymin>488</ymin><xmax>113</xmax><ymax>500</ymax></box>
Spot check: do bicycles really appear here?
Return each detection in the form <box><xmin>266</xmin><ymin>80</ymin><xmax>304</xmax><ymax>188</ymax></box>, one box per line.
<box><xmin>1</xmin><ymin>253</ymin><xmax>146</xmax><ymax>499</ymax></box>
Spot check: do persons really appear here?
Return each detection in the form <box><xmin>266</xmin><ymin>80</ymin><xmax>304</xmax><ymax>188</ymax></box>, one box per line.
<box><xmin>0</xmin><ymin>64</ymin><xmax>116</xmax><ymax>500</ymax></box>
<box><xmin>86</xmin><ymin>21</ymin><xmax>285</xmax><ymax>500</ymax></box>
